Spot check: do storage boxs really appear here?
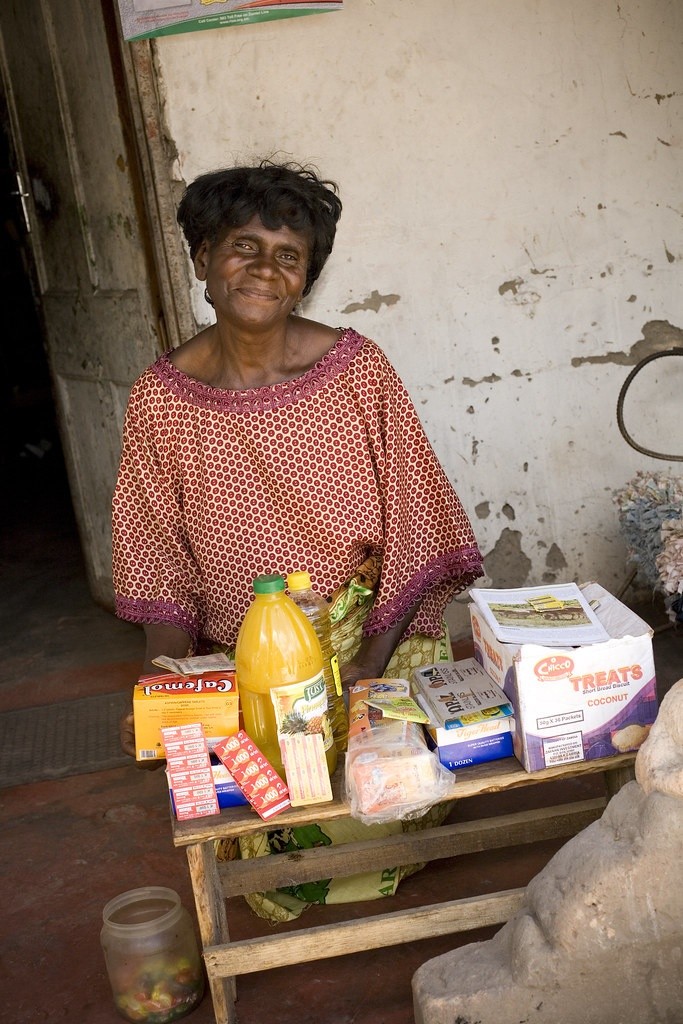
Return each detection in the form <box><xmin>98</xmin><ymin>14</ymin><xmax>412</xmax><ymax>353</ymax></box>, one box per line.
<box><xmin>132</xmin><ymin>671</ymin><xmax>239</xmax><ymax>761</ymax></box>
<box><xmin>431</xmin><ymin>732</ymin><xmax>515</xmax><ymax>770</ymax></box>
<box><xmin>415</xmin><ymin>690</ymin><xmax>516</xmax><ymax>746</ymax></box>
<box><xmin>468</xmin><ymin>582</ymin><xmax>659</xmax><ymax>774</ymax></box>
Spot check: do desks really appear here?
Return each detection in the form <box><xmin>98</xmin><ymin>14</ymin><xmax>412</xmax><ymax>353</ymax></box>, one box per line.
<box><xmin>168</xmin><ymin>753</ymin><xmax>636</xmax><ymax>1024</ymax></box>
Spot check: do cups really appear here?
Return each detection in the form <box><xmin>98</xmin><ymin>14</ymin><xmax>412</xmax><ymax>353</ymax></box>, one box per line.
<box><xmin>98</xmin><ymin>884</ymin><xmax>201</xmax><ymax>1024</ymax></box>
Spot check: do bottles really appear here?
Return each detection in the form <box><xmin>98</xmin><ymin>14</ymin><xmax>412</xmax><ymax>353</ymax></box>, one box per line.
<box><xmin>287</xmin><ymin>572</ymin><xmax>350</xmax><ymax>752</ymax></box>
<box><xmin>235</xmin><ymin>575</ymin><xmax>336</xmax><ymax>786</ymax></box>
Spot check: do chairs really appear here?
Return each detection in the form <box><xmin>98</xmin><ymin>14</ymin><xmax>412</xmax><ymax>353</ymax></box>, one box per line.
<box><xmin>612</xmin><ymin>349</ymin><xmax>683</xmax><ymax>633</ymax></box>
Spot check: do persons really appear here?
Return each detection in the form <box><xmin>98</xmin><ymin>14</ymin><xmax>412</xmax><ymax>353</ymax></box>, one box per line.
<box><xmin>111</xmin><ymin>150</ymin><xmax>483</xmax><ymax>927</ymax></box>
